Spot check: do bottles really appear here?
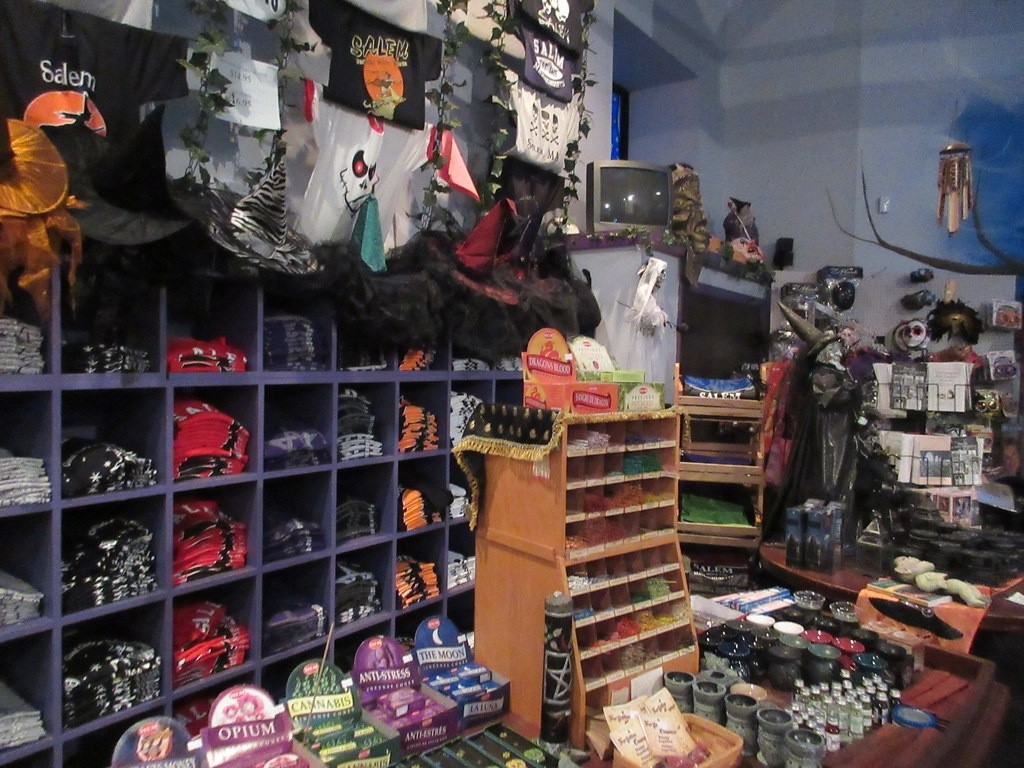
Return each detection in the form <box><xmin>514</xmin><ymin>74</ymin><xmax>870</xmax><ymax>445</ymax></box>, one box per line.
<box><xmin>793</xmin><ymin>668</ymin><xmax>902</xmax><ymax>752</ymax></box>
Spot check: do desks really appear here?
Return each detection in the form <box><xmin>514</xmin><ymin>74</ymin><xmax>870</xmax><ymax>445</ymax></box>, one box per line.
<box><xmin>398</xmin><ymin>628</ymin><xmax>1011</xmax><ymax>768</ymax></box>
<box><xmin>761</xmin><ymin>540</ymin><xmax>1024</xmax><ymax>634</ymax></box>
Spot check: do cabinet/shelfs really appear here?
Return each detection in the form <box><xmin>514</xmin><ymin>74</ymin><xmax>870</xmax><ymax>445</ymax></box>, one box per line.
<box><xmin>1</xmin><ymin>228</ymin><xmax>772</xmax><ymax>768</ymax></box>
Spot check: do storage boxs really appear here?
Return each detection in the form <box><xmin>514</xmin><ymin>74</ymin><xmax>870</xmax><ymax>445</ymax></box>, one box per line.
<box><xmin>111</xmin><ymin>616</ymin><xmax>511</xmax><ymax>768</ymax></box>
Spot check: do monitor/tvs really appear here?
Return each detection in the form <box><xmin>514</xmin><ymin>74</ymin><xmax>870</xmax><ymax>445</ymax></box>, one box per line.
<box><xmin>586</xmin><ymin>160</ymin><xmax>672</xmax><ymax>234</ymax></box>
